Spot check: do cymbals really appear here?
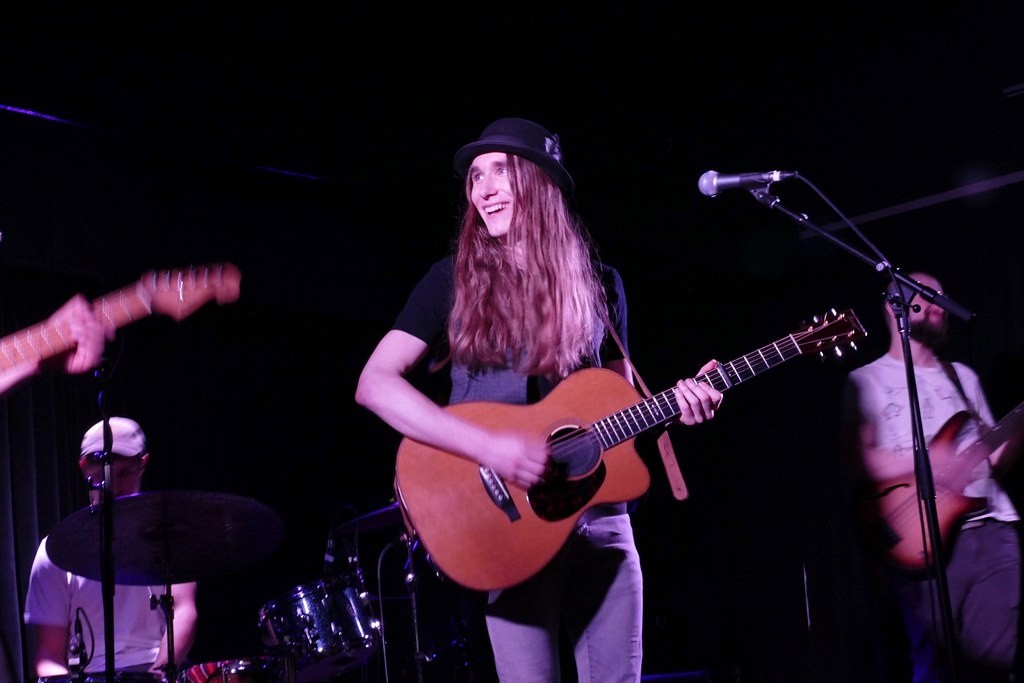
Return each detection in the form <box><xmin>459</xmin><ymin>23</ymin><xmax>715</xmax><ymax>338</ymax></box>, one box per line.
<box><xmin>340</xmin><ymin>501</ymin><xmax>404</xmax><ymax>533</ymax></box>
<box><xmin>47</xmin><ymin>490</ymin><xmax>277</xmax><ymax>587</ymax></box>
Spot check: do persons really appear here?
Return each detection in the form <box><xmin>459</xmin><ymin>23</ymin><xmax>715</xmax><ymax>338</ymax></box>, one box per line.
<box><xmin>24</xmin><ymin>417</ymin><xmax>199</xmax><ymax>681</ymax></box>
<box><xmin>355</xmin><ymin>118</ymin><xmax>725</xmax><ymax>683</ymax></box>
<box><xmin>854</xmin><ymin>273</ymin><xmax>1022</xmax><ymax>682</ymax></box>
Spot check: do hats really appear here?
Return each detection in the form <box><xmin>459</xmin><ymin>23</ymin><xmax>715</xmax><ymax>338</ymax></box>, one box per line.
<box><xmin>456</xmin><ymin>116</ymin><xmax>575</xmax><ymax>195</ymax></box>
<box><xmin>80</xmin><ymin>418</ymin><xmax>144</xmax><ymax>457</ymax></box>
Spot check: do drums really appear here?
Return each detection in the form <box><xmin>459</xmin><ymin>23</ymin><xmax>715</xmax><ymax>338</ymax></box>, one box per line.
<box><xmin>258</xmin><ymin>572</ymin><xmax>385</xmax><ymax>681</ymax></box>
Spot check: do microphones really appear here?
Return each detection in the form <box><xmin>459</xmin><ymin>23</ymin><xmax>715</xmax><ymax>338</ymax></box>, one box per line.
<box><xmin>698</xmin><ymin>170</ymin><xmax>796</xmax><ymax>197</ymax></box>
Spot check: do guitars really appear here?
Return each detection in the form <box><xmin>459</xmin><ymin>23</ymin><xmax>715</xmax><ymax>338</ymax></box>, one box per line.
<box><xmin>394</xmin><ymin>308</ymin><xmax>866</xmax><ymax>601</ymax></box>
<box><xmin>867</xmin><ymin>397</ymin><xmax>1024</xmax><ymax>577</ymax></box>
